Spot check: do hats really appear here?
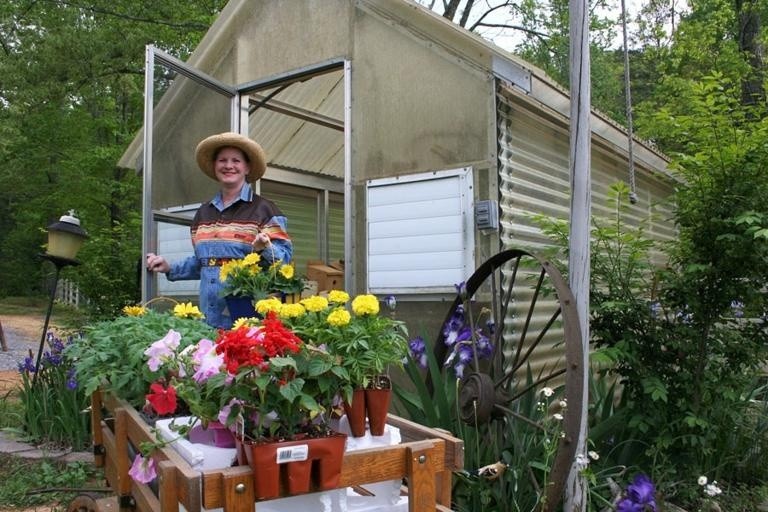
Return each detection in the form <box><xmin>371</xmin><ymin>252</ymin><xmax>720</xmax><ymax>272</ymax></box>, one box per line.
<box><xmin>194</xmin><ymin>132</ymin><xmax>266</xmax><ymax>184</ymax></box>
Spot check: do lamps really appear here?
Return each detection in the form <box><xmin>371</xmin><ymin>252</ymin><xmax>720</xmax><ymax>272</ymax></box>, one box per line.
<box><xmin>22</xmin><ymin>207</ymin><xmax>90</xmax><ymax>434</ymax></box>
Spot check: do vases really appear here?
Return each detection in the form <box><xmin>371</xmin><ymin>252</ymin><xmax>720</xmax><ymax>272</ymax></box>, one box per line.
<box><xmin>234</xmin><ymin>422</ymin><xmax>349</xmax><ymax>501</ymax></box>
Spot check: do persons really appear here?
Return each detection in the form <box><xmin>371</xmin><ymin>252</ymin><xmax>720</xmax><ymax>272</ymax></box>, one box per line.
<box><xmin>145</xmin><ymin>132</ymin><xmax>294</xmax><ymax>332</ymax></box>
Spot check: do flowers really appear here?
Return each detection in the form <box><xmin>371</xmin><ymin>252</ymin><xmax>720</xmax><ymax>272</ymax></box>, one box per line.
<box><xmin>124</xmin><ymin>235</ymin><xmax>411</xmax><ymax>488</ymax></box>
